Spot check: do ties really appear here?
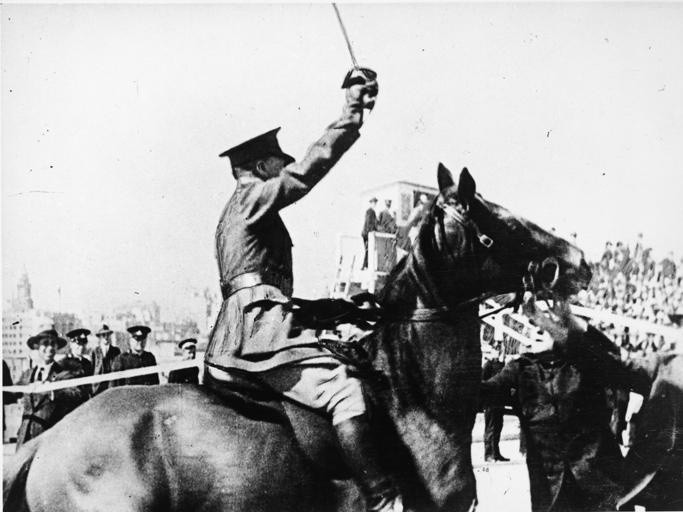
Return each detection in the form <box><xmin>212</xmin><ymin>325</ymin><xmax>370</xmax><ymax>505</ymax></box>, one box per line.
<box><xmin>37</xmin><ymin>367</ymin><xmax>44</xmax><ymax>380</ymax></box>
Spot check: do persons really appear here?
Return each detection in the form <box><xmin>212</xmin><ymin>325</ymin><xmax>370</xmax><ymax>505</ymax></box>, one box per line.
<box><xmin>203</xmin><ymin>66</ymin><xmax>402</xmax><ymax>505</ymax></box>
<box><xmin>360</xmin><ymin>193</ymin><xmax>430</xmax><ymax>269</ymax></box>
<box><xmin>483</xmin><ymin>234</ymin><xmax>682</xmax><ymax>449</ymax></box>
<box><xmin>479</xmin><ymin>336</ymin><xmax>512</xmax><ymax>462</ymax></box>
<box><xmin>589</xmin><ymin>305</ymin><xmax>682</xmax><ymax>511</ymax></box>
<box><xmin>474</xmin><ymin>291</ymin><xmax>651</xmax><ymax>510</ymax></box>
<box><xmin>1</xmin><ymin>321</ymin><xmax>202</xmax><ymax>450</ymax></box>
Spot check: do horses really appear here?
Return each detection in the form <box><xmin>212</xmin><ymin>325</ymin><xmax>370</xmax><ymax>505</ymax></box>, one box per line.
<box><xmin>3</xmin><ymin>163</ymin><xmax>593</xmax><ymax>512</ymax></box>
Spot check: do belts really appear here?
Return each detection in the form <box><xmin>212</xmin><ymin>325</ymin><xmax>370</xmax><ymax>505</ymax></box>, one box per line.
<box><xmin>220</xmin><ymin>269</ymin><xmax>294</xmax><ymax>301</ymax></box>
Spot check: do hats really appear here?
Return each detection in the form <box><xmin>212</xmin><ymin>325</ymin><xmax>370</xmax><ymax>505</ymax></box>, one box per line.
<box><xmin>95</xmin><ymin>324</ymin><xmax>113</xmax><ymax>337</ymax></box>
<box><xmin>219</xmin><ymin>127</ymin><xmax>295</xmax><ymax>167</ymax></box>
<box><xmin>65</xmin><ymin>329</ymin><xmax>91</xmax><ymax>346</ymax></box>
<box><xmin>178</xmin><ymin>338</ymin><xmax>197</xmax><ymax>350</ymax></box>
<box><xmin>27</xmin><ymin>330</ymin><xmax>68</xmax><ymax>350</ymax></box>
<box><xmin>127</xmin><ymin>326</ymin><xmax>150</xmax><ymax>341</ymax></box>
